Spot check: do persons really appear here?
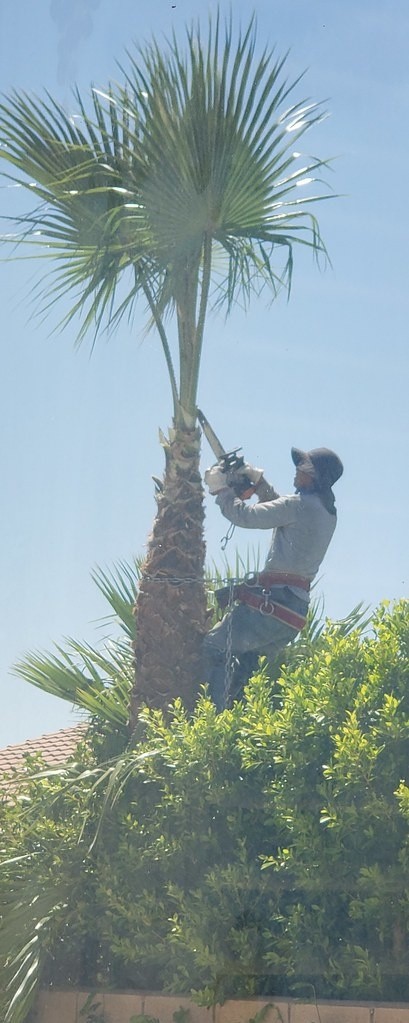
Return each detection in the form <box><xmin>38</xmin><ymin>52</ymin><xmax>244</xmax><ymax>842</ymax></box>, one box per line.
<box><xmin>202</xmin><ymin>447</ymin><xmax>344</xmax><ymax>712</ymax></box>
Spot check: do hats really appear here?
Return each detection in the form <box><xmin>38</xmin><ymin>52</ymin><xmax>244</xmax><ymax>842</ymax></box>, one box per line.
<box><xmin>291</xmin><ymin>446</ymin><xmax>343</xmax><ymax>515</ymax></box>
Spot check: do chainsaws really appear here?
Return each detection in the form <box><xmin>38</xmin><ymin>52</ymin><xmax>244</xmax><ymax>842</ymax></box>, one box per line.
<box><xmin>196</xmin><ymin>406</ymin><xmax>256</xmax><ymax>502</ymax></box>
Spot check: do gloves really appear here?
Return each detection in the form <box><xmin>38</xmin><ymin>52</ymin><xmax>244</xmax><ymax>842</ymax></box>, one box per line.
<box><xmin>230</xmin><ymin>460</ymin><xmax>264</xmax><ymax>485</ymax></box>
<box><xmin>204</xmin><ymin>461</ymin><xmax>228</xmax><ymax>495</ymax></box>
